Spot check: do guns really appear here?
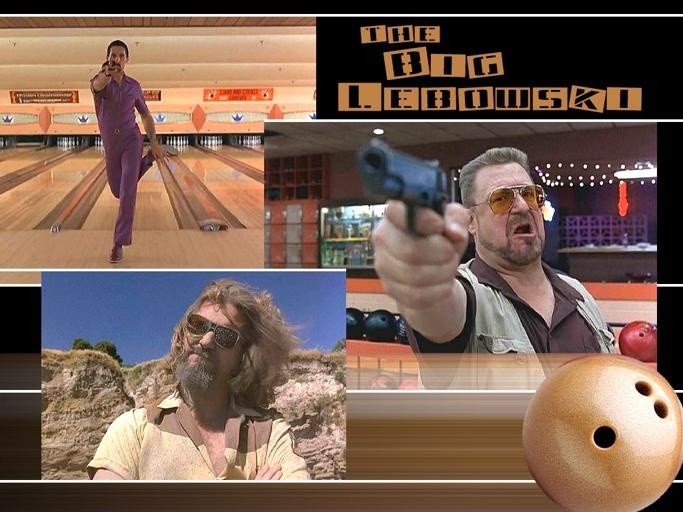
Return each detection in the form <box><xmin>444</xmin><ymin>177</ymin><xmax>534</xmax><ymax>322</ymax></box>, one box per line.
<box><xmin>355</xmin><ymin>137</ymin><xmax>447</xmax><ymax>238</ymax></box>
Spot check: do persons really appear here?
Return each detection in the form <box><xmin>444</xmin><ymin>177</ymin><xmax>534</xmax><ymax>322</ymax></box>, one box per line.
<box><xmin>370</xmin><ymin>147</ymin><xmax>620</xmax><ymax>355</ymax></box>
<box><xmin>90</xmin><ymin>40</ymin><xmax>178</xmax><ymax>265</ymax></box>
<box><xmin>85</xmin><ymin>278</ymin><xmax>312</xmax><ymax>480</ymax></box>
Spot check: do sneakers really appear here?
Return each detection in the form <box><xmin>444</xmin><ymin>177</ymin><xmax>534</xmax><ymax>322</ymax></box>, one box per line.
<box><xmin>148</xmin><ymin>145</ymin><xmax>178</xmax><ymax>157</ymax></box>
<box><xmin>110</xmin><ymin>248</ymin><xmax>122</xmax><ymax>263</ymax></box>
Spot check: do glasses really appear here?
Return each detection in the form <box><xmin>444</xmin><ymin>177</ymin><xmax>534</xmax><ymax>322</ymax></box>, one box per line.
<box><xmin>185</xmin><ymin>313</ymin><xmax>249</xmax><ymax>351</ymax></box>
<box><xmin>472</xmin><ymin>184</ymin><xmax>545</xmax><ymax>215</ymax></box>
